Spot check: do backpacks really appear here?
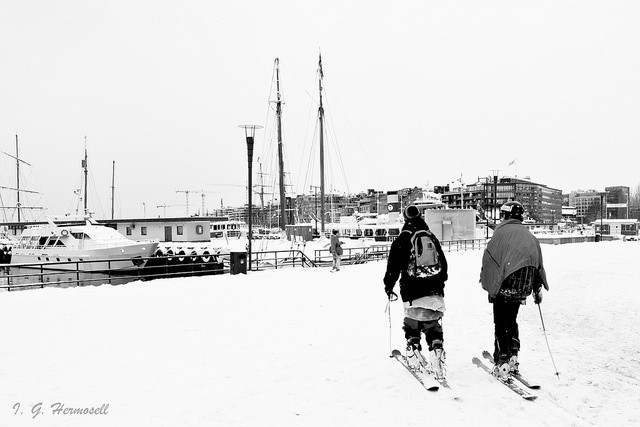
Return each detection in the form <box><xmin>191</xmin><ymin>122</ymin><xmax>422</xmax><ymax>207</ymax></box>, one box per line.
<box><xmin>402</xmin><ymin>229</ymin><xmax>442</xmax><ymax>278</ymax></box>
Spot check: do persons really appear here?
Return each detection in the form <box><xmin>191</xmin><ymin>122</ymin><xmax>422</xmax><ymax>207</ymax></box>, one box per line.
<box><xmin>479</xmin><ymin>200</ymin><xmax>549</xmax><ymax>383</ymax></box>
<box><xmin>329</xmin><ymin>229</ymin><xmax>345</xmax><ymax>272</ymax></box>
<box><xmin>383</xmin><ymin>204</ymin><xmax>448</xmax><ymax>383</ymax></box>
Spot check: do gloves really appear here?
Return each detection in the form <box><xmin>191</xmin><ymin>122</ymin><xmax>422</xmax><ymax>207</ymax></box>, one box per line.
<box><xmin>385</xmin><ymin>286</ymin><xmax>394</xmax><ymax>297</ymax></box>
<box><xmin>532</xmin><ymin>286</ymin><xmax>542</xmax><ymax>303</ymax></box>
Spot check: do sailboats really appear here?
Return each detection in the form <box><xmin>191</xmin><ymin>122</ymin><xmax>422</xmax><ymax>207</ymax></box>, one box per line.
<box><xmin>208</xmin><ymin>52</ymin><xmax>405</xmax><ymax>262</ymax></box>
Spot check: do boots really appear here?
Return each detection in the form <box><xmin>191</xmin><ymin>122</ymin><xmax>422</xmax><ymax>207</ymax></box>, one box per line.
<box><xmin>430</xmin><ymin>343</ymin><xmax>447</xmax><ymax>380</ymax></box>
<box><xmin>406</xmin><ymin>336</ymin><xmax>420</xmax><ymax>371</ymax></box>
<box><xmin>508</xmin><ymin>352</ymin><xmax>520</xmax><ymax>376</ymax></box>
<box><xmin>492</xmin><ymin>354</ymin><xmax>513</xmax><ymax>383</ymax></box>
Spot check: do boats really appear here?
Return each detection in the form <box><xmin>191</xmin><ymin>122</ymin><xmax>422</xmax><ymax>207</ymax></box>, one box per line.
<box><xmin>7</xmin><ymin>211</ymin><xmax>159</xmax><ymax>272</ymax></box>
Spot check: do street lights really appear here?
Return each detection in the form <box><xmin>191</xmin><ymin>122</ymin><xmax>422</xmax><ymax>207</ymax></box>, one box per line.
<box><xmin>598</xmin><ymin>192</ymin><xmax>606</xmax><ymax>241</ymax></box>
<box><xmin>490</xmin><ymin>169</ymin><xmax>502</xmax><ymax>230</ymax></box>
<box><xmin>235</xmin><ymin>123</ymin><xmax>265</xmax><ymax>272</ymax></box>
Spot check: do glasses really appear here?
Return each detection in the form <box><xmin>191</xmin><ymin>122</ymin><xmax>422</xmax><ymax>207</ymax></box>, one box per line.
<box><xmin>499</xmin><ymin>214</ymin><xmax>507</xmax><ymax>219</ymax></box>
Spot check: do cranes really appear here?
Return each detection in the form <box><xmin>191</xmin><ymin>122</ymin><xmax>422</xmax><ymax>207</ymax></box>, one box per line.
<box><xmin>137</xmin><ymin>201</ymin><xmax>153</xmax><ymax>218</ymax></box>
<box><xmin>192</xmin><ymin>187</ymin><xmax>222</xmax><ymax>216</ymax></box>
<box><xmin>176</xmin><ymin>187</ymin><xmax>201</xmax><ymax>215</ymax></box>
<box><xmin>157</xmin><ymin>202</ymin><xmax>185</xmax><ymax>216</ymax></box>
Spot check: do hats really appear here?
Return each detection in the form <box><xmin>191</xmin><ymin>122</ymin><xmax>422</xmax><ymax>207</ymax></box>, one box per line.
<box><xmin>403</xmin><ymin>204</ymin><xmax>422</xmax><ymax>221</ymax></box>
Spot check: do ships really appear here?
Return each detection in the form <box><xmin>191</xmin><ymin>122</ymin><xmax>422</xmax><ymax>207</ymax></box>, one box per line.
<box><xmin>0</xmin><ymin>214</ymin><xmax>230</xmax><ymax>281</ymax></box>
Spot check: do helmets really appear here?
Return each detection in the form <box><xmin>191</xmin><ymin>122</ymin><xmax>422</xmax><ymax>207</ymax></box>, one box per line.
<box><xmin>500</xmin><ymin>201</ymin><xmax>524</xmax><ymax>214</ymax></box>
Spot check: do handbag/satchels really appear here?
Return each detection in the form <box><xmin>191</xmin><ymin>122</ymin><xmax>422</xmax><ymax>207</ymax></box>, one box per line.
<box><xmin>336</xmin><ymin>247</ymin><xmax>343</xmax><ymax>255</ymax></box>
